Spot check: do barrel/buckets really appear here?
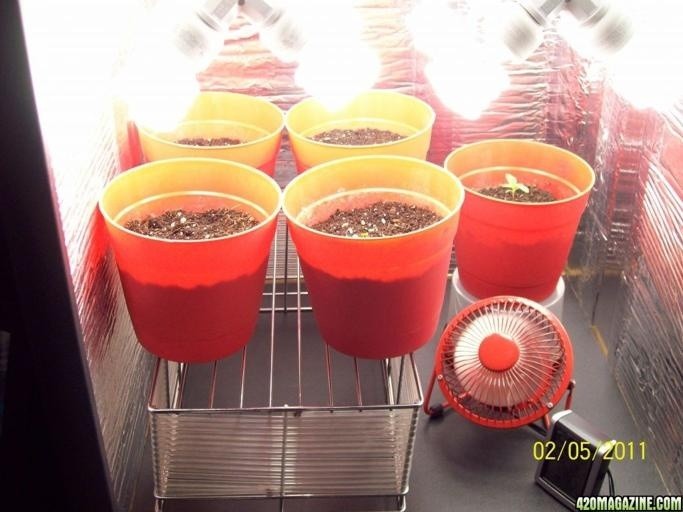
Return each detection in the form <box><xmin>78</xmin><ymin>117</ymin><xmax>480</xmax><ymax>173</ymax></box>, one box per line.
<box><xmin>441</xmin><ymin>267</ymin><xmax>566</xmax><ymax>406</ymax></box>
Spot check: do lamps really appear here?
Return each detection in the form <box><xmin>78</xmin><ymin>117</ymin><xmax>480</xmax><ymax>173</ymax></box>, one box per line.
<box><xmin>112</xmin><ymin>1</ymin><xmax>383</xmax><ymax>130</ymax></box>
<box><xmin>418</xmin><ymin>0</ymin><xmax>678</xmax><ymax>124</ymax></box>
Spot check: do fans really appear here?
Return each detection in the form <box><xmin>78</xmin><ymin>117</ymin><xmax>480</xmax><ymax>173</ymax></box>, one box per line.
<box><xmin>422</xmin><ymin>290</ymin><xmax>576</xmax><ymax>439</ymax></box>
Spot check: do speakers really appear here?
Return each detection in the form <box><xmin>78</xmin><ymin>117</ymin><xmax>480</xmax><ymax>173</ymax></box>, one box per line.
<box><xmin>535</xmin><ymin>410</ymin><xmax>617</xmax><ymax>512</ymax></box>
